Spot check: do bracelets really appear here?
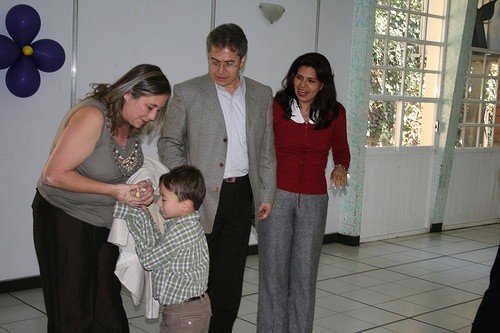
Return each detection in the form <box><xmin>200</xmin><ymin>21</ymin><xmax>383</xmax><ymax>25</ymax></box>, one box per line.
<box><xmin>335</xmin><ymin>164</ymin><xmax>347</xmax><ymax>174</ymax></box>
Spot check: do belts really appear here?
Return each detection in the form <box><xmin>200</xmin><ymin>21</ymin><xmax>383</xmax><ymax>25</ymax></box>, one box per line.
<box><xmin>222</xmin><ymin>177</ymin><xmax>249</xmax><ymax>184</ymax></box>
<box><xmin>182</xmin><ymin>293</ymin><xmax>206</xmax><ymax>303</ymax></box>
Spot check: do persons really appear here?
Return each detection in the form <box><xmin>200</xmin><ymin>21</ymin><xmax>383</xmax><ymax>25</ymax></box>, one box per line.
<box><xmin>113</xmin><ymin>165</ymin><xmax>212</xmax><ymax>333</ymax></box>
<box><xmin>256</xmin><ymin>53</ymin><xmax>351</xmax><ymax>333</ymax></box>
<box><xmin>156</xmin><ymin>24</ymin><xmax>278</xmax><ymax>333</ymax></box>
<box><xmin>32</xmin><ymin>63</ymin><xmax>171</xmax><ymax>333</ymax></box>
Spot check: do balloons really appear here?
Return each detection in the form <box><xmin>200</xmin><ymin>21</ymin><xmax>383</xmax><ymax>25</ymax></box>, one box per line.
<box><xmin>0</xmin><ymin>4</ymin><xmax>65</xmax><ymax>98</ymax></box>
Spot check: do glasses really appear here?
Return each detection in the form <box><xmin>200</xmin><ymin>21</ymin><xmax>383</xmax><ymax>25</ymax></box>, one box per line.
<box><xmin>208</xmin><ymin>59</ymin><xmax>236</xmax><ymax>68</ymax></box>
